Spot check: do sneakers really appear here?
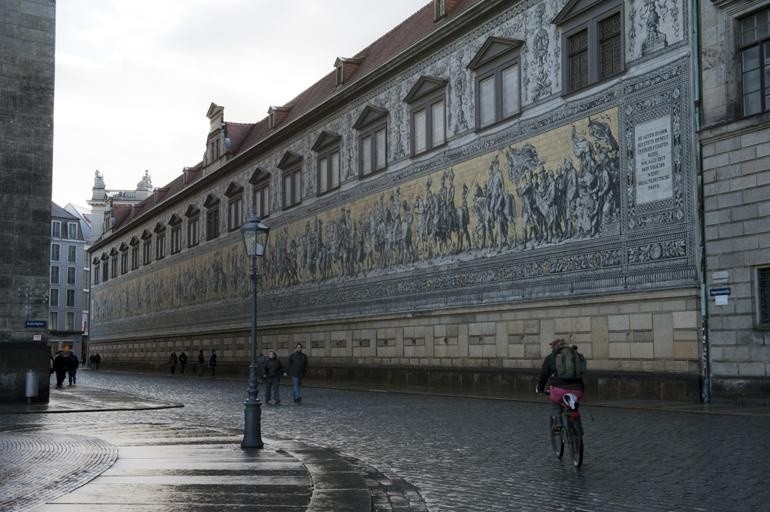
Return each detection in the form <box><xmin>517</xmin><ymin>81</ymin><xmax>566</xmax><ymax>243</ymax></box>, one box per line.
<box><xmin>294</xmin><ymin>397</ymin><xmax>301</xmax><ymax>401</ymax></box>
<box><xmin>275</xmin><ymin>400</ymin><xmax>281</xmax><ymax>405</ymax></box>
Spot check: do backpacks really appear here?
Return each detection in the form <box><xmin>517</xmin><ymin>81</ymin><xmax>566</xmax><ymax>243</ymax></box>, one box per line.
<box><xmin>555</xmin><ymin>345</ymin><xmax>586</xmax><ymax>379</ymax></box>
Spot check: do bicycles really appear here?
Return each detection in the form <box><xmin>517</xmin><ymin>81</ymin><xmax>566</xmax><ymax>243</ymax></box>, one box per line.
<box><xmin>534</xmin><ymin>382</ymin><xmax>585</xmax><ymax>469</ymax></box>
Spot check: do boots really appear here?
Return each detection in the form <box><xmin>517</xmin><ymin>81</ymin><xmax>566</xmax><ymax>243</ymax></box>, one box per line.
<box><xmin>552</xmin><ymin>416</ymin><xmax>562</xmax><ymax>432</ymax></box>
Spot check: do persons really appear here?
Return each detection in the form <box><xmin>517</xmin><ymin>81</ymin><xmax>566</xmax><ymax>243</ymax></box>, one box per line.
<box><xmin>66</xmin><ymin>351</ymin><xmax>79</xmax><ymax>385</ymax></box>
<box><xmin>207</xmin><ymin>348</ymin><xmax>217</xmax><ymax>376</ymax></box>
<box><xmin>81</xmin><ymin>352</ymin><xmax>87</xmax><ymax>368</ymax></box>
<box><xmin>178</xmin><ymin>351</ymin><xmax>187</xmax><ymax>374</ymax></box>
<box><xmin>536</xmin><ymin>337</ymin><xmax>585</xmax><ymax>435</ymax></box>
<box><xmin>285</xmin><ymin>342</ymin><xmax>308</xmax><ymax>403</ymax></box>
<box><xmin>53</xmin><ymin>350</ymin><xmax>67</xmax><ymax>386</ymax></box>
<box><xmin>197</xmin><ymin>349</ymin><xmax>205</xmax><ymax>376</ymax></box>
<box><xmin>167</xmin><ymin>351</ymin><xmax>178</xmax><ymax>374</ymax></box>
<box><xmin>95</xmin><ymin>352</ymin><xmax>101</xmax><ymax>369</ymax></box>
<box><xmin>48</xmin><ymin>352</ymin><xmax>59</xmax><ymax>377</ymax></box>
<box><xmin>90</xmin><ymin>354</ymin><xmax>96</xmax><ymax>369</ymax></box>
<box><xmin>260</xmin><ymin>350</ymin><xmax>287</xmax><ymax>404</ymax></box>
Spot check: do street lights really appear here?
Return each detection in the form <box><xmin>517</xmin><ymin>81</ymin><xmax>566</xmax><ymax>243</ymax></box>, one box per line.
<box><xmin>238</xmin><ymin>206</ymin><xmax>271</xmax><ymax>447</ymax></box>
